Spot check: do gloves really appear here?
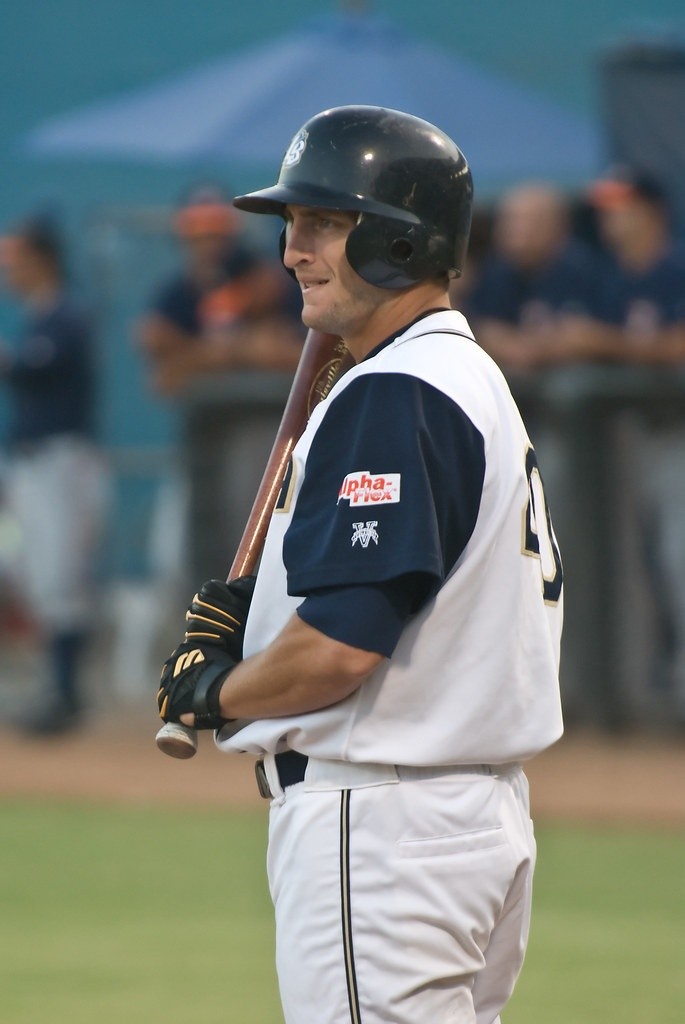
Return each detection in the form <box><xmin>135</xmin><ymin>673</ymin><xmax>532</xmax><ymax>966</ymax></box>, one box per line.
<box><xmin>156</xmin><ymin>643</ymin><xmax>237</xmax><ymax>729</ymax></box>
<box><xmin>185</xmin><ymin>575</ymin><xmax>257</xmax><ymax>663</ymax></box>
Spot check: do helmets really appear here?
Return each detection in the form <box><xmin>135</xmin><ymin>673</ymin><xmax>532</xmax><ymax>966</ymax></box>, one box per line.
<box><xmin>231</xmin><ymin>106</ymin><xmax>473</xmax><ymax>289</ymax></box>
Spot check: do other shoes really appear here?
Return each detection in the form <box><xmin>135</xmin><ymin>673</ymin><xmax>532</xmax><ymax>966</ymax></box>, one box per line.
<box><xmin>30</xmin><ymin>704</ymin><xmax>86</xmax><ymax>736</ymax></box>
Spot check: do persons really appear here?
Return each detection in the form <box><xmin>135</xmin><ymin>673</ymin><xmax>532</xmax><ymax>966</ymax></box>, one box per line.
<box><xmin>0</xmin><ymin>164</ymin><xmax>684</xmax><ymax>736</ymax></box>
<box><xmin>160</xmin><ymin>105</ymin><xmax>566</xmax><ymax>1024</ymax></box>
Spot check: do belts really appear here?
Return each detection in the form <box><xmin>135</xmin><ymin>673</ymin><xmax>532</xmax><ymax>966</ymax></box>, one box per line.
<box><xmin>255</xmin><ymin>749</ymin><xmax>309</xmax><ymax>798</ymax></box>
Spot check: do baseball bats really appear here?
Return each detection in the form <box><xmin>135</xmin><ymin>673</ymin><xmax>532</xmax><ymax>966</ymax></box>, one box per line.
<box><xmin>154</xmin><ymin>322</ymin><xmax>350</xmax><ymax>762</ymax></box>
